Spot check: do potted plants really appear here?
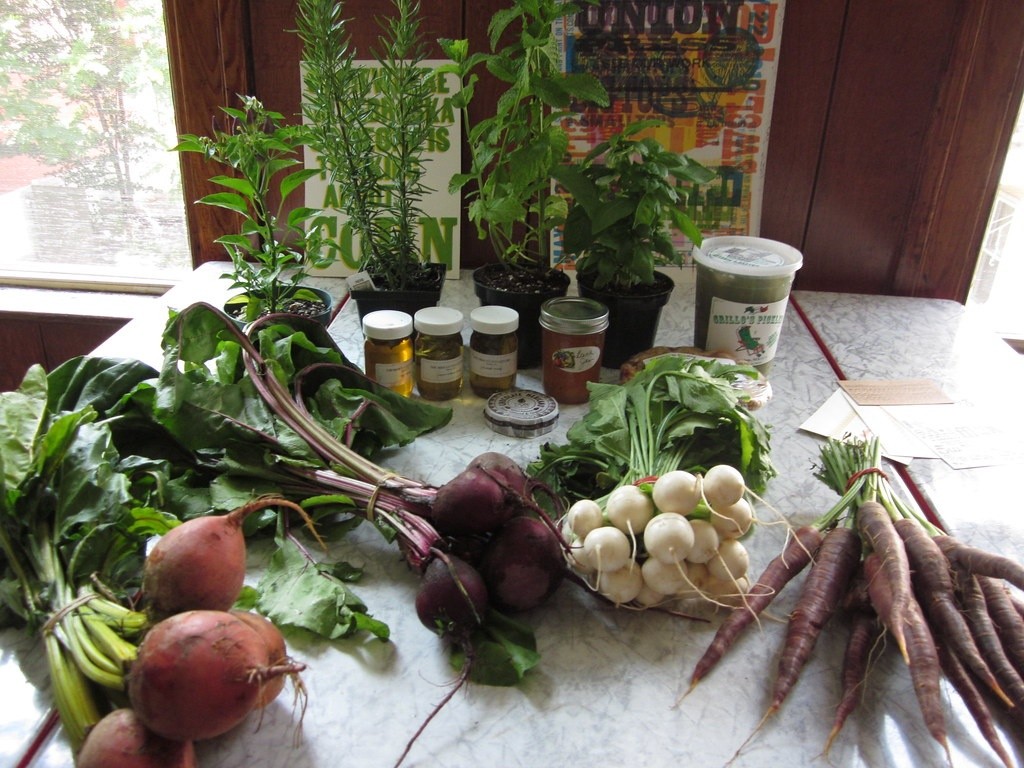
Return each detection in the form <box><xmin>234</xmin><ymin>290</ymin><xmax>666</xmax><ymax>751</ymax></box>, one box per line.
<box><xmin>169</xmin><ymin>93</ymin><xmax>331</xmax><ymax>329</ymax></box>
<box><xmin>282</xmin><ymin>0</ymin><xmax>452</xmax><ymax>343</ymax></box>
<box><xmin>435</xmin><ymin>0</ymin><xmax>612</xmax><ymax>373</ymax></box>
<box><xmin>546</xmin><ymin>120</ymin><xmax>715</xmax><ymax>372</ymax></box>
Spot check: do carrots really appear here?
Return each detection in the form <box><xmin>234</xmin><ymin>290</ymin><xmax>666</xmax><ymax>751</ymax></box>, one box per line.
<box><xmin>671</xmin><ymin>501</ymin><xmax>1024</xmax><ymax>768</ymax></box>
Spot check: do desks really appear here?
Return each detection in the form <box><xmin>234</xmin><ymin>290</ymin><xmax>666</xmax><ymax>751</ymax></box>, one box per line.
<box><xmin>0</xmin><ymin>261</ymin><xmax>1023</xmax><ymax>768</ymax></box>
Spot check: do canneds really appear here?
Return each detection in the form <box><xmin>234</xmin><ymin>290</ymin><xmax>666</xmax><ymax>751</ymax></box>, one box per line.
<box><xmin>362</xmin><ymin>306</ymin><xmax>520</xmax><ymax>401</ymax></box>
<box><xmin>537</xmin><ymin>296</ymin><xmax>609</xmax><ymax>405</ymax></box>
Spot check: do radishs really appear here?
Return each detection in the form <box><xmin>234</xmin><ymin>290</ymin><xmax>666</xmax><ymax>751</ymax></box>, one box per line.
<box><xmin>561</xmin><ymin>463</ymin><xmax>753</xmax><ymax>606</ymax></box>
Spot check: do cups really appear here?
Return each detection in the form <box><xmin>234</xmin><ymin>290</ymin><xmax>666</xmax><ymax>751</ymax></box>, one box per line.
<box><xmin>539</xmin><ymin>296</ymin><xmax>609</xmax><ymax>404</ymax></box>
<box><xmin>692</xmin><ymin>236</ymin><xmax>803</xmax><ymax>379</ymax></box>
